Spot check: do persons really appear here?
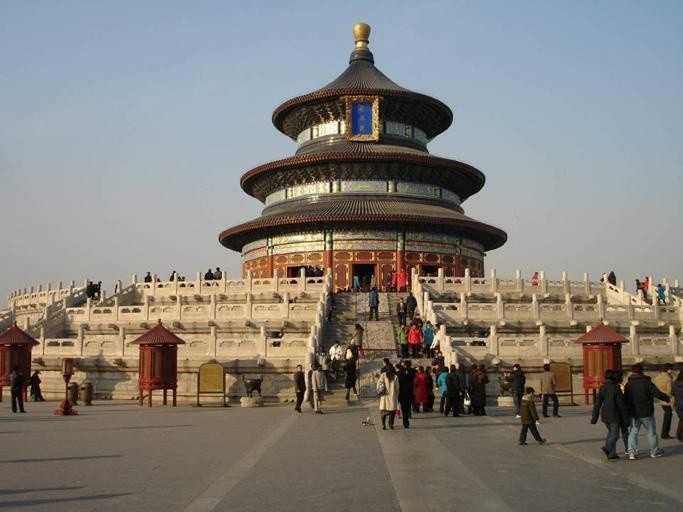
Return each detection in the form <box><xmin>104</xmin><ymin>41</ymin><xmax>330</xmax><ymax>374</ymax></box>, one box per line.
<box><xmin>503</xmin><ymin>362</ymin><xmax>683</xmax><ymax>460</ymax></box>
<box><xmin>599</xmin><ymin>271</ymin><xmax>617</xmax><ymax>291</ymax></box>
<box><xmin>30</xmin><ymin>370</ymin><xmax>45</xmax><ymax>402</ymax></box>
<box><xmin>635</xmin><ymin>277</ymin><xmax>667</xmax><ymax>305</ymax></box>
<box><xmin>293</xmin><ymin>266</ymin><xmax>489</xmax><ymax>430</ymax></box>
<box><xmin>530</xmin><ymin>271</ymin><xmax>538</xmax><ymax>287</ymax></box>
<box><xmin>86</xmin><ymin>266</ymin><xmax>222</xmax><ymax>300</ymax></box>
<box><xmin>9</xmin><ymin>363</ymin><xmax>26</xmax><ymax>413</ymax></box>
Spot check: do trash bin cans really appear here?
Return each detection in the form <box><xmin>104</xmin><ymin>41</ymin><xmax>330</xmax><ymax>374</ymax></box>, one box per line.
<box><xmin>67</xmin><ymin>382</ymin><xmax>79</xmax><ymax>406</ymax></box>
<box><xmin>79</xmin><ymin>383</ymin><xmax>94</xmax><ymax>406</ymax></box>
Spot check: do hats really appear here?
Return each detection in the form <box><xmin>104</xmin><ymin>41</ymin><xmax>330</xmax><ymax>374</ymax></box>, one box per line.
<box><xmin>630</xmin><ymin>365</ymin><xmax>643</xmax><ymax>372</ymax></box>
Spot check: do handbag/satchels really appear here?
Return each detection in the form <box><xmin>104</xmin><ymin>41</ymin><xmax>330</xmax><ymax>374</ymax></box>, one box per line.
<box><xmin>377</xmin><ymin>383</ymin><xmax>386</xmax><ymax>395</ymax></box>
<box><xmin>464</xmin><ymin>399</ymin><xmax>472</xmax><ymax>406</ymax></box>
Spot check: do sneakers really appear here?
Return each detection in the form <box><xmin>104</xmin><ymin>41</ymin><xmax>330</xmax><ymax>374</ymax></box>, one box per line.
<box><xmin>609</xmin><ymin>456</ymin><xmax>620</xmax><ymax>460</ymax></box>
<box><xmin>625</xmin><ymin>448</ymin><xmax>639</xmax><ymax>460</ymax></box>
<box><xmin>650</xmin><ymin>449</ymin><xmax>664</xmax><ymax>457</ymax></box>
